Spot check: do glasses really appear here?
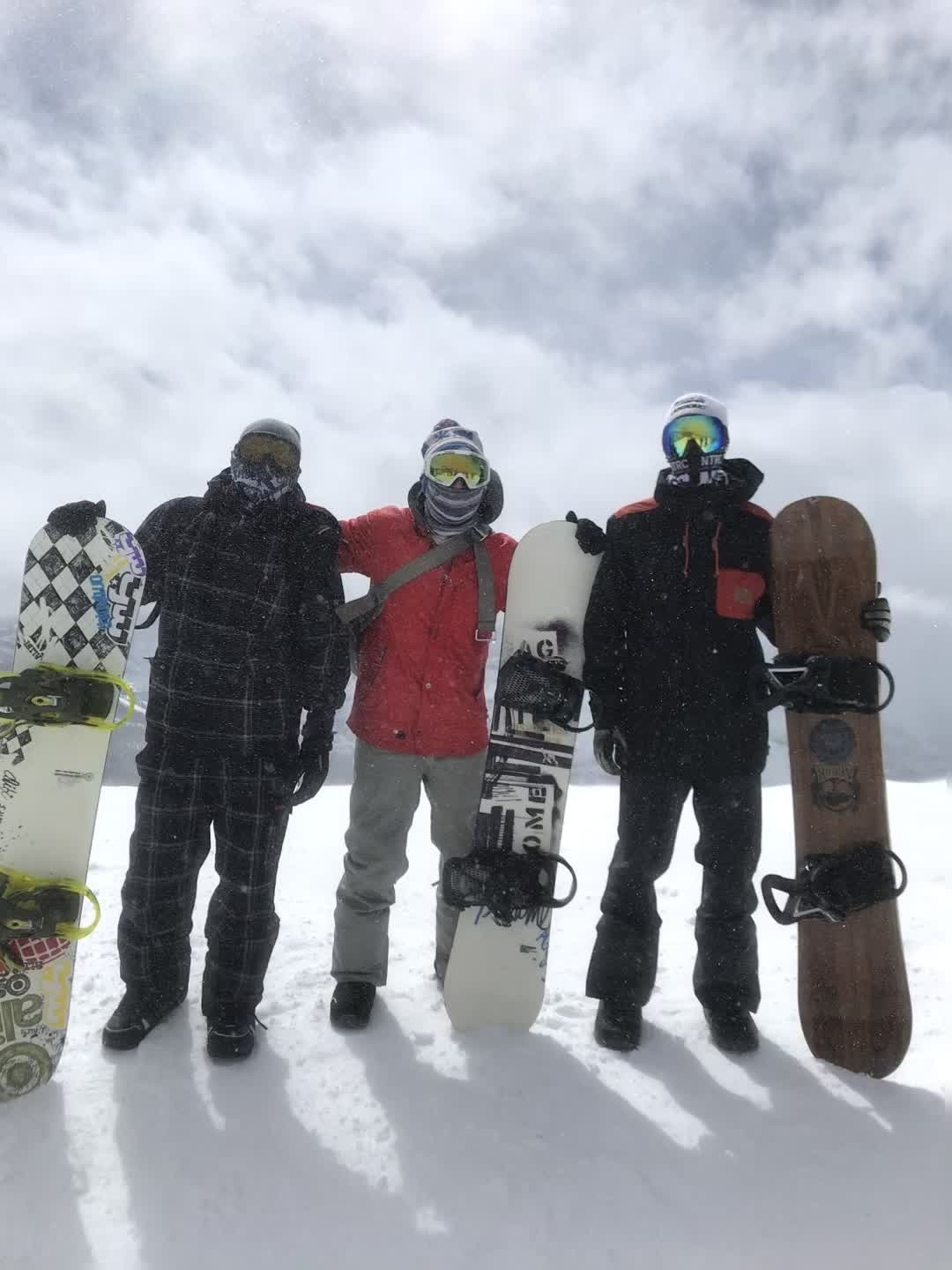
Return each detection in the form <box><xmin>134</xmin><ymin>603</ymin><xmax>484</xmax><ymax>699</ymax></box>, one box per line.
<box><xmin>662</xmin><ymin>413</ymin><xmax>727</xmax><ymax>460</ymax></box>
<box><xmin>424</xmin><ymin>452</ymin><xmax>491</xmax><ymax>489</ymax></box>
<box><xmin>235</xmin><ymin>432</ymin><xmax>300</xmax><ymax>476</ymax></box>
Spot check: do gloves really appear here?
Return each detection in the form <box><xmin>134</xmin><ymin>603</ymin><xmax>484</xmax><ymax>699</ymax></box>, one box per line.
<box><xmin>593</xmin><ymin>725</ymin><xmax>626</xmax><ymax>775</ymax></box>
<box><xmin>567</xmin><ymin>511</ymin><xmax>607</xmax><ymax>556</ymax></box>
<box><xmin>861</xmin><ymin>582</ymin><xmax>891</xmax><ymax>642</ymax></box>
<box><xmin>47</xmin><ymin>500</ymin><xmax>106</xmax><ymax>536</ymax></box>
<box><xmin>281</xmin><ymin>728</ymin><xmax>334</xmax><ymax>806</ymax></box>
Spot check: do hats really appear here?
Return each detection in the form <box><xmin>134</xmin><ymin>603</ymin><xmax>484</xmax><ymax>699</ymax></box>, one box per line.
<box><xmin>422</xmin><ymin>418</ymin><xmax>488</xmax><ymax>523</ymax></box>
<box><xmin>238</xmin><ymin>418</ymin><xmax>301</xmax><ymax>453</ymax></box>
<box><xmin>666</xmin><ymin>393</ymin><xmax>726</xmax><ymax>426</ymax></box>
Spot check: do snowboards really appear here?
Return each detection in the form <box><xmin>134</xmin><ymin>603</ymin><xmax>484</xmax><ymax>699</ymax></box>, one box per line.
<box><xmin>766</xmin><ymin>490</ymin><xmax>917</xmax><ymax>1082</ymax></box>
<box><xmin>439</xmin><ymin>517</ymin><xmax>609</xmax><ymax>1040</ymax></box>
<box><xmin>1</xmin><ymin>515</ymin><xmax>154</xmax><ymax>1105</ymax></box>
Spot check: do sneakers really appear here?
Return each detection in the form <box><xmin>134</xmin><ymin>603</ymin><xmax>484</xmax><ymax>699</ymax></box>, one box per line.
<box><xmin>595</xmin><ymin>999</ymin><xmax>641</xmax><ymax>1049</ymax></box>
<box><xmin>329</xmin><ymin>982</ymin><xmax>375</xmax><ymax>1027</ymax></box>
<box><xmin>103</xmin><ymin>988</ymin><xmax>177</xmax><ymax>1050</ymax></box>
<box><xmin>705</xmin><ymin>1005</ymin><xmax>759</xmax><ymax>1052</ymax></box>
<box><xmin>205</xmin><ymin>1002</ymin><xmax>256</xmax><ymax>1059</ymax></box>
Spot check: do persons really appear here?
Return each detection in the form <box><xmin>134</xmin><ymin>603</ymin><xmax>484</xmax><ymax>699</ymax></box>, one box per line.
<box><xmin>329</xmin><ymin>418</ymin><xmax>606</xmax><ymax>1029</ymax></box>
<box><xmin>580</xmin><ymin>392</ymin><xmax>892</xmax><ymax>1052</ymax></box>
<box><xmin>47</xmin><ymin>417</ymin><xmax>350</xmax><ymax>1059</ymax></box>
